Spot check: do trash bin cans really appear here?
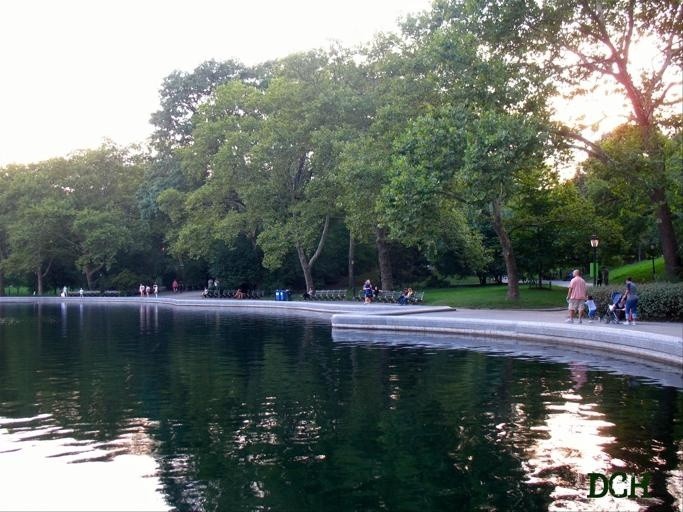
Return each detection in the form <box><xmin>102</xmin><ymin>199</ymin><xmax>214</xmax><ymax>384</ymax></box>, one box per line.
<box><xmin>274</xmin><ymin>290</ymin><xmax>292</xmax><ymax>301</ymax></box>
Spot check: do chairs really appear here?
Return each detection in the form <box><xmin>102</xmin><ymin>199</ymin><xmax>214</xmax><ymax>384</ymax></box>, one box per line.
<box><xmin>360</xmin><ymin>290</ymin><xmax>424</xmax><ymax>306</ymax></box>
<box><xmin>203</xmin><ymin>289</ymin><xmax>265</xmax><ymax>299</ymax></box>
<box><xmin>304</xmin><ymin>290</ymin><xmax>348</xmax><ymax>301</ymax></box>
<box><xmin>60</xmin><ymin>287</ymin><xmax>122</xmax><ymax>297</ymax></box>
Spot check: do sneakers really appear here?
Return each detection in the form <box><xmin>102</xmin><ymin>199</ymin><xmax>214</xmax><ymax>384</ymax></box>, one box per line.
<box><xmin>564</xmin><ymin>318</ymin><xmax>638</xmax><ymax>326</ymax></box>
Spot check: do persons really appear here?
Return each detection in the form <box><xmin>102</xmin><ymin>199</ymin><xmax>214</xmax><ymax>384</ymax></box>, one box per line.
<box><xmin>63</xmin><ymin>285</ymin><xmax>69</xmax><ymax>297</ymax></box>
<box><xmin>233</xmin><ymin>289</ymin><xmax>247</xmax><ymax>299</ymax></box>
<box><xmin>138</xmin><ymin>283</ymin><xmax>158</xmax><ymax>297</ymax></box>
<box><xmin>565</xmin><ymin>264</ymin><xmax>640</xmax><ymax>325</ymax></box>
<box><xmin>362</xmin><ymin>278</ymin><xmax>416</xmax><ymax>306</ymax></box>
<box><xmin>302</xmin><ymin>287</ymin><xmax>314</xmax><ymax>300</ymax></box>
<box><xmin>201</xmin><ymin>277</ymin><xmax>220</xmax><ymax>298</ymax></box>
<box><xmin>171</xmin><ymin>279</ymin><xmax>184</xmax><ymax>295</ymax></box>
<box><xmin>79</xmin><ymin>288</ymin><xmax>84</xmax><ymax>297</ymax></box>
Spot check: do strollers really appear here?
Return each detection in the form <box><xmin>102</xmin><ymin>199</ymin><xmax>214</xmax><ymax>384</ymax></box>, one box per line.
<box><xmin>604</xmin><ymin>291</ymin><xmax>627</xmax><ymax>324</ymax></box>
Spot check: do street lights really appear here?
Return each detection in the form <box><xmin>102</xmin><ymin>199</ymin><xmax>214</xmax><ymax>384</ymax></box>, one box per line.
<box><xmin>648</xmin><ymin>243</ymin><xmax>657</xmax><ymax>273</ymax></box>
<box><xmin>590</xmin><ymin>233</ymin><xmax>599</xmax><ymax>286</ymax></box>
<box><xmin>350</xmin><ymin>256</ymin><xmax>359</xmax><ymax>297</ymax></box>
<box><xmin>179</xmin><ymin>263</ymin><xmax>185</xmax><ymax>282</ymax></box>
